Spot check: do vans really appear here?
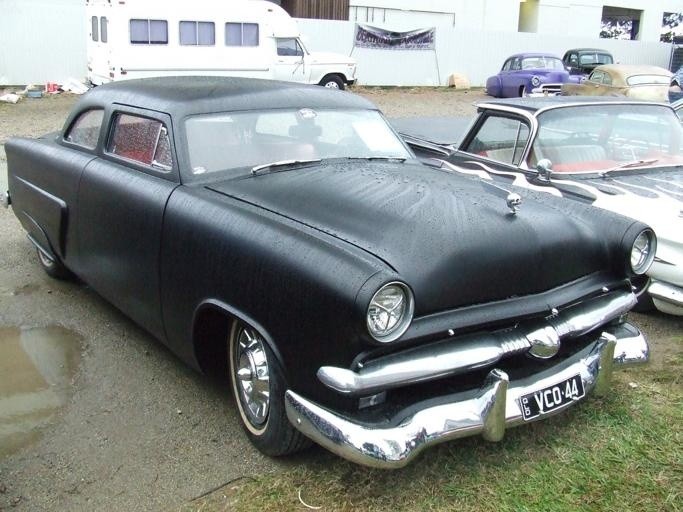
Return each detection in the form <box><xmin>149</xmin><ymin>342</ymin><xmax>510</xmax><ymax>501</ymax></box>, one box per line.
<box><xmin>85</xmin><ymin>0</ymin><xmax>359</xmax><ymax>91</ymax></box>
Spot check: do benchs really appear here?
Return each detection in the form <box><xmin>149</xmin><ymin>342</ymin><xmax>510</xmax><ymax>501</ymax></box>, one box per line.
<box><xmin>481</xmin><ymin>144</ymin><xmax>606</xmax><ymax>165</ymax></box>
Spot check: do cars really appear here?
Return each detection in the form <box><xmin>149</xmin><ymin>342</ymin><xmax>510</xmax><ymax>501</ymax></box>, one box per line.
<box><xmin>483</xmin><ymin>53</ymin><xmax>581</xmax><ymax>98</ymax></box>
<box><xmin>562</xmin><ymin>65</ymin><xmax>675</xmax><ymax>104</ymax></box>
<box><xmin>3</xmin><ymin>63</ymin><xmax>658</xmax><ymax>470</ymax></box>
<box><xmin>561</xmin><ymin>49</ymin><xmax>616</xmax><ymax>78</ymax></box>
<box><xmin>390</xmin><ymin>96</ymin><xmax>683</xmax><ymax>315</ymax></box>
<box><xmin>669</xmin><ymin>67</ymin><xmax>683</xmax><ymax>103</ymax></box>
